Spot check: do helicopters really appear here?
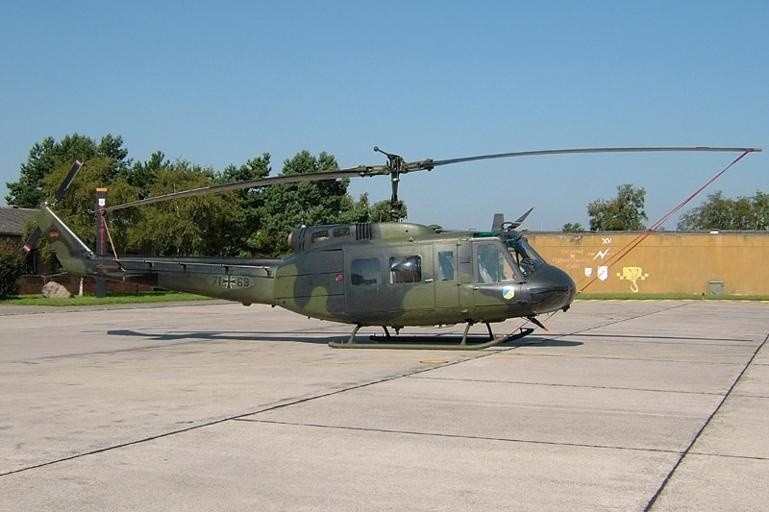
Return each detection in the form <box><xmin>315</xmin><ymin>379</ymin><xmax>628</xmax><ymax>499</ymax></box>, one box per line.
<box><xmin>23</xmin><ymin>147</ymin><xmax>765</xmax><ymax>351</ymax></box>
